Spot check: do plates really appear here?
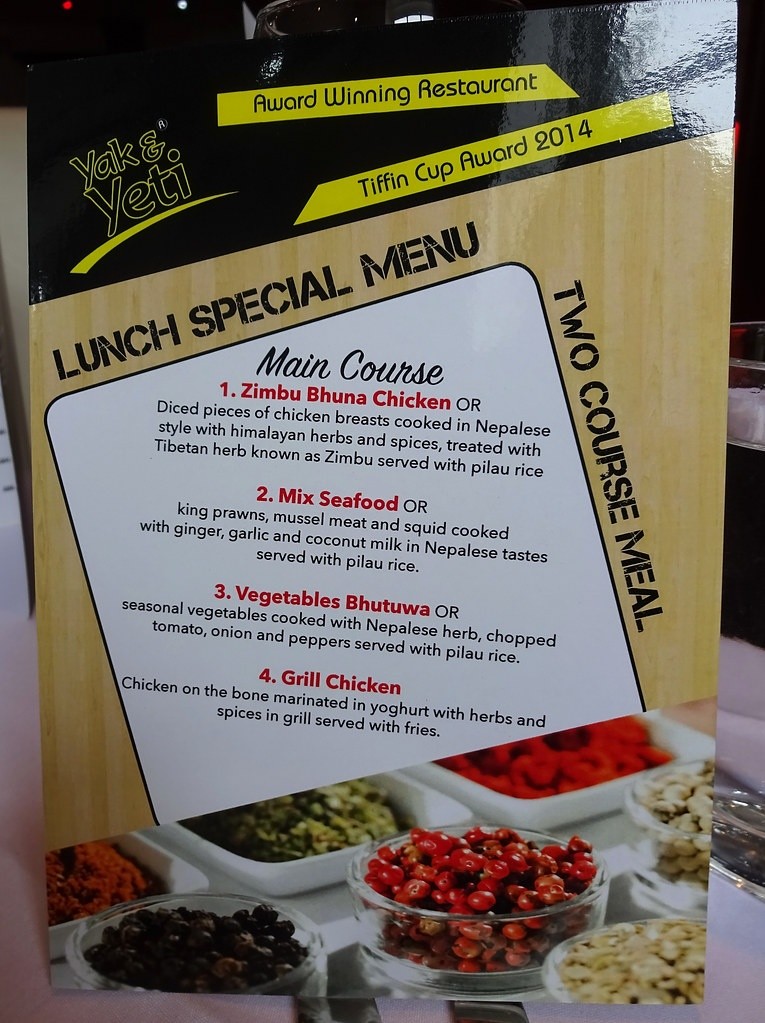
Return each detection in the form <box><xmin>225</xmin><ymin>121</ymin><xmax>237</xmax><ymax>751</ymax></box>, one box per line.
<box><xmin>406</xmin><ymin>711</ymin><xmax>715</xmax><ymax>826</ymax></box>
<box><xmin>149</xmin><ymin>769</ymin><xmax>476</xmax><ymax>898</ymax></box>
<box><xmin>47</xmin><ymin>831</ymin><xmax>210</xmax><ymax>960</ymax></box>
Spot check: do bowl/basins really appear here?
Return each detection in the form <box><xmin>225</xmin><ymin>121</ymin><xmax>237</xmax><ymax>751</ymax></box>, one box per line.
<box><xmin>624</xmin><ymin>759</ymin><xmax>714</xmax><ymax>891</ymax></box>
<box><xmin>348</xmin><ymin>825</ymin><xmax>610</xmax><ymax>1002</ymax></box>
<box><xmin>66</xmin><ymin>892</ymin><xmax>328</xmax><ymax>999</ymax></box>
<box><xmin>542</xmin><ymin>918</ymin><xmax>706</xmax><ymax>1005</ymax></box>
